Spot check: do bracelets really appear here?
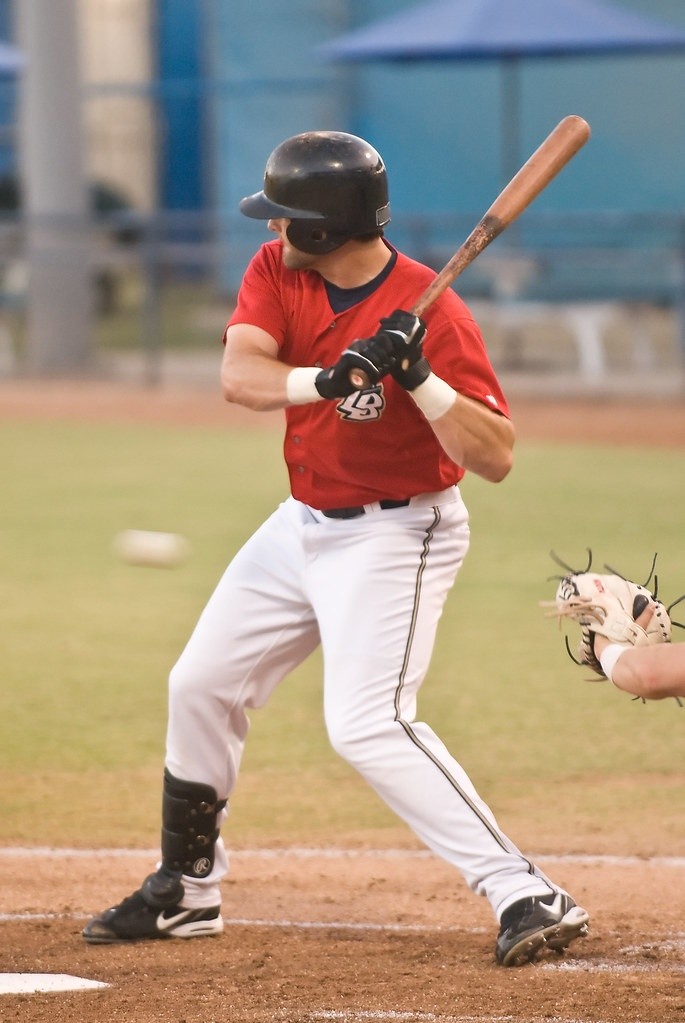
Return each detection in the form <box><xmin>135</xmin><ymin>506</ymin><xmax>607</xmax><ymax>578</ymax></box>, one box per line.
<box><xmin>406</xmin><ymin>371</ymin><xmax>458</xmax><ymax>422</ymax></box>
<box><xmin>598</xmin><ymin>643</ymin><xmax>630</xmax><ymax>689</ymax></box>
<box><xmin>284</xmin><ymin>367</ymin><xmax>324</xmax><ymax>405</ymax></box>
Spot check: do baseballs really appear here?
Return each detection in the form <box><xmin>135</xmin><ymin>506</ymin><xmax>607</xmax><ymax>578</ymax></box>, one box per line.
<box><xmin>117</xmin><ymin>528</ymin><xmax>181</xmax><ymax>573</ymax></box>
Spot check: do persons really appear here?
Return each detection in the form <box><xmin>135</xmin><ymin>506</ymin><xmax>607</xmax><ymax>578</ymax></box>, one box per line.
<box><xmin>593</xmin><ymin>602</ymin><xmax>685</xmax><ymax>700</ymax></box>
<box><xmin>78</xmin><ymin>130</ymin><xmax>591</xmax><ymax>968</ymax></box>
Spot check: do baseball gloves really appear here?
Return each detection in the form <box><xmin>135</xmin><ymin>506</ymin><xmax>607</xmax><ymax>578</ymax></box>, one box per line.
<box><xmin>546</xmin><ymin>545</ymin><xmax>685</xmax><ymax>688</ymax></box>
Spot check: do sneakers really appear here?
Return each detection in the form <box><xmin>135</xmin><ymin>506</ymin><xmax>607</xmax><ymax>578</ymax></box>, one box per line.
<box><xmin>81</xmin><ymin>871</ymin><xmax>227</xmax><ymax>945</ymax></box>
<box><xmin>495</xmin><ymin>894</ymin><xmax>592</xmax><ymax>966</ymax></box>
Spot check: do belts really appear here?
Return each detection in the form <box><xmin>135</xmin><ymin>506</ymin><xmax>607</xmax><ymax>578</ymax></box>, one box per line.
<box><xmin>318</xmin><ymin>498</ymin><xmax>410</xmax><ymax>520</ymax></box>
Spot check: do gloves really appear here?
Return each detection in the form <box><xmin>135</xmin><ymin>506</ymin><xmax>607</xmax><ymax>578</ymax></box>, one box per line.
<box><xmin>315</xmin><ymin>308</ymin><xmax>432</xmax><ymax>401</ymax></box>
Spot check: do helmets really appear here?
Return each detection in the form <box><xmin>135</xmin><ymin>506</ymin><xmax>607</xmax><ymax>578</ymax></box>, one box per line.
<box><xmin>236</xmin><ymin>131</ymin><xmax>392</xmax><ymax>253</ymax></box>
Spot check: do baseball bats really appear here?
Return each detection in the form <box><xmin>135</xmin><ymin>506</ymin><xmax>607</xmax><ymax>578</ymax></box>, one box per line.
<box><xmin>348</xmin><ymin>112</ymin><xmax>590</xmax><ymax>391</ymax></box>
